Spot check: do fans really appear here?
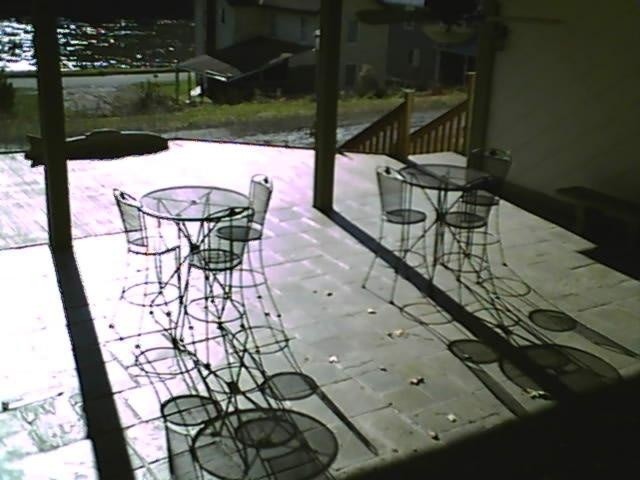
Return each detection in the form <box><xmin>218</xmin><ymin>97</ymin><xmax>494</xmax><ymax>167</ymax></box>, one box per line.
<box><xmin>355</xmin><ymin>1</ymin><xmax>563</xmax><ymax>50</ymax></box>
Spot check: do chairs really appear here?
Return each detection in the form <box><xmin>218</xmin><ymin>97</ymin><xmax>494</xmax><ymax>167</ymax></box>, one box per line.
<box><xmin>362</xmin><ymin>146</ymin><xmax>511</xmax><ymax>303</ymax></box>
<box><xmin>112</xmin><ymin>174</ymin><xmax>290</xmax><ymax>364</ymax></box>
